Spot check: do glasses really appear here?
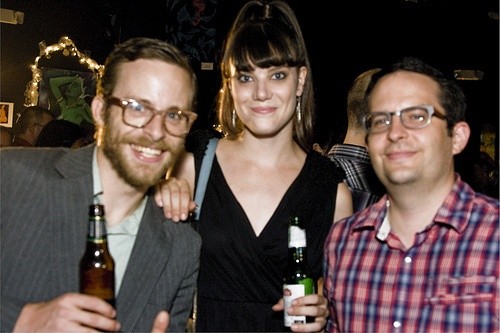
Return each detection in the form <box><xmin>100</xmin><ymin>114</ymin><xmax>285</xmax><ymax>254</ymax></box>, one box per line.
<box><xmin>362</xmin><ymin>105</ymin><xmax>447</xmax><ymax>135</ymax></box>
<box><xmin>105</xmin><ymin>97</ymin><xmax>199</xmax><ymax>137</ymax></box>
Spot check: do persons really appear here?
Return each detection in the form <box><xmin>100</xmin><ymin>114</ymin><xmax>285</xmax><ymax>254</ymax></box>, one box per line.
<box><xmin>324</xmin><ymin>68</ymin><xmax>388</xmax><ymax>214</ymax></box>
<box><xmin>0</xmin><ymin>37</ymin><xmax>202</xmax><ymax>333</ymax></box>
<box><xmin>323</xmin><ymin>58</ymin><xmax>500</xmax><ymax>333</ymax></box>
<box><xmin>34</xmin><ymin>118</ymin><xmax>90</xmax><ymax>149</ymax></box>
<box><xmin>153</xmin><ymin>1</ymin><xmax>354</xmax><ymax>333</ymax></box>
<box><xmin>9</xmin><ymin>106</ymin><xmax>57</xmax><ymax>149</ymax></box>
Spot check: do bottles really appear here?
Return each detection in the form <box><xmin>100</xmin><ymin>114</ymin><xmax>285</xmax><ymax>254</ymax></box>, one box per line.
<box><xmin>283</xmin><ymin>215</ymin><xmax>314</xmax><ymax>331</ymax></box>
<box><xmin>80</xmin><ymin>204</ymin><xmax>116</xmax><ymax>333</ymax></box>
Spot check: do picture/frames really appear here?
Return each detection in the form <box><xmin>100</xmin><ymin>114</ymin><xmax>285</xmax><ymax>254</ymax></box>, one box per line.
<box><xmin>0</xmin><ymin>101</ymin><xmax>14</xmax><ymax>128</ymax></box>
